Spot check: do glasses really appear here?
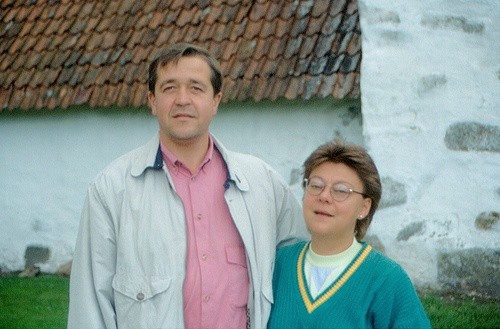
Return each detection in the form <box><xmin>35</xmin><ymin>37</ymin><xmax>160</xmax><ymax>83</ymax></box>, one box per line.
<box><xmin>303</xmin><ymin>176</ymin><xmax>367</xmax><ymax>203</ymax></box>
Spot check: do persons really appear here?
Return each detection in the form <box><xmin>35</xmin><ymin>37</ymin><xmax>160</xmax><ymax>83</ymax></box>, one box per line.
<box><xmin>66</xmin><ymin>42</ymin><xmax>312</xmax><ymax>329</ymax></box>
<box><xmin>265</xmin><ymin>138</ymin><xmax>433</xmax><ymax>329</ymax></box>
